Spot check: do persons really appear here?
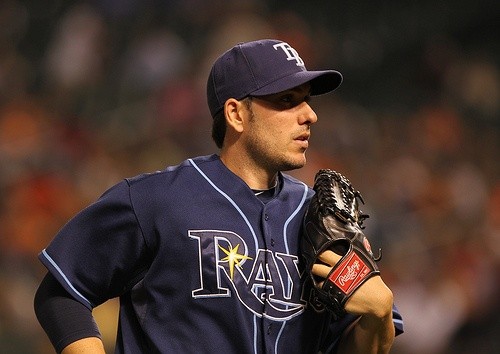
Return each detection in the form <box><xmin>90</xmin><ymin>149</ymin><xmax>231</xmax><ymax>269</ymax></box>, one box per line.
<box><xmin>34</xmin><ymin>37</ymin><xmax>405</xmax><ymax>354</ymax></box>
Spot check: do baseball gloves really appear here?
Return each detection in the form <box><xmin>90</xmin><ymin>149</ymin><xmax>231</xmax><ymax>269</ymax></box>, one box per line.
<box><xmin>297</xmin><ymin>168</ymin><xmax>382</xmax><ymax>309</ymax></box>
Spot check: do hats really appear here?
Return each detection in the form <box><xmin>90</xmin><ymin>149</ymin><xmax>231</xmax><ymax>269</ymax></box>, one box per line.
<box><xmin>207</xmin><ymin>39</ymin><xmax>343</xmax><ymax>118</ymax></box>
